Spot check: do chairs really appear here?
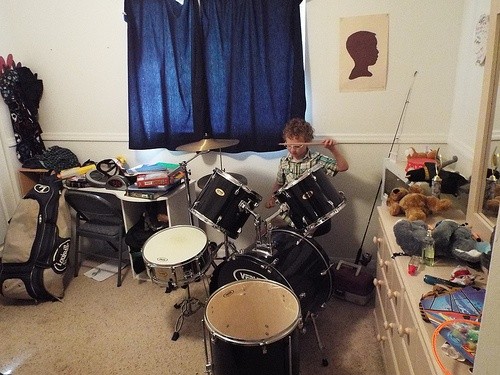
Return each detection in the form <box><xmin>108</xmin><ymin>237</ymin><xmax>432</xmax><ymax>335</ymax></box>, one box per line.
<box><xmin>64</xmin><ymin>191</ymin><xmax>130</xmax><ymax>287</ymax></box>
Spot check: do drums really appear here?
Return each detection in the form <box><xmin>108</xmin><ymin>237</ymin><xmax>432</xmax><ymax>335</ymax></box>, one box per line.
<box><xmin>188</xmin><ymin>167</ymin><xmax>263</xmax><ymax>240</ymax></box>
<box><xmin>271</xmin><ymin>163</ymin><xmax>347</xmax><ymax>234</ymax></box>
<box><xmin>203</xmin><ymin>278</ymin><xmax>302</xmax><ymax>375</ymax></box>
<box><xmin>140</xmin><ymin>224</ymin><xmax>214</xmax><ymax>287</ymax></box>
<box><xmin>213</xmin><ymin>226</ymin><xmax>334</xmax><ymax>325</ymax></box>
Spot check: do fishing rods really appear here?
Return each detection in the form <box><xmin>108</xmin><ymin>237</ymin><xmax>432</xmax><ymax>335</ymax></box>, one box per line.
<box><xmin>354</xmin><ymin>70</ymin><xmax>419</xmax><ymax>268</ymax></box>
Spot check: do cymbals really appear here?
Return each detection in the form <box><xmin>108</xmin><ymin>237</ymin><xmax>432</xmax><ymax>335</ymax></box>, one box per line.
<box><xmin>197</xmin><ymin>172</ymin><xmax>248</xmax><ymax>189</ymax></box>
<box><xmin>175</xmin><ymin>136</ymin><xmax>239</xmax><ymax>152</ymax></box>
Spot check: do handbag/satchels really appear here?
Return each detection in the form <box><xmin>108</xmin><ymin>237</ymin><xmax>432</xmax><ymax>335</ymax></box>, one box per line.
<box><xmin>0</xmin><ymin>174</ymin><xmax>72</xmax><ymax>303</ymax></box>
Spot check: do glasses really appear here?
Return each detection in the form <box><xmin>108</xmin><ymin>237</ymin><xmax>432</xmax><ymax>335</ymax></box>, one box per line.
<box><xmin>284</xmin><ymin>142</ymin><xmax>303</xmax><ymax>149</ymax></box>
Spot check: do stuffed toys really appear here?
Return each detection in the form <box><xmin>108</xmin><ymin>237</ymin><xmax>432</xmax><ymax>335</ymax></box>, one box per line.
<box><xmin>386</xmin><ymin>147</ymin><xmax>487</xmax><ymax>266</ymax></box>
<box><xmin>483</xmin><ymin>167</ymin><xmax>500</xmax><ymax>219</ymax></box>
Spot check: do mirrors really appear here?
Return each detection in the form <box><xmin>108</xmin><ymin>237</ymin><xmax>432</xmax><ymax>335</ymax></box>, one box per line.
<box><xmin>466</xmin><ymin>15</ymin><xmax>500</xmax><ymax>244</ymax></box>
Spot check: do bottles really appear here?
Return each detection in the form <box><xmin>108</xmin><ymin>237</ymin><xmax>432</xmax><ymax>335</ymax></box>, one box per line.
<box><xmin>408</xmin><ymin>255</ymin><xmax>422</xmax><ymax>275</ymax></box>
<box><xmin>422</xmin><ymin>229</ymin><xmax>435</xmax><ymax>266</ymax></box>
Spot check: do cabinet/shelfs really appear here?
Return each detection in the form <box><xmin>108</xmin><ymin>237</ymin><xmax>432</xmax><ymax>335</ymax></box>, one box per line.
<box><xmin>373</xmin><ymin>205</ymin><xmax>500</xmax><ymax>375</ymax></box>
<box><xmin>18</xmin><ymin>168</ymin><xmax>192</xmax><ymax>283</ymax></box>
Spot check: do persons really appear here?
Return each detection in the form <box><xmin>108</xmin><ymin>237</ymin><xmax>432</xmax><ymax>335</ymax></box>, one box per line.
<box><xmin>264</xmin><ymin>119</ymin><xmax>349</xmax><ymax>208</ymax></box>
